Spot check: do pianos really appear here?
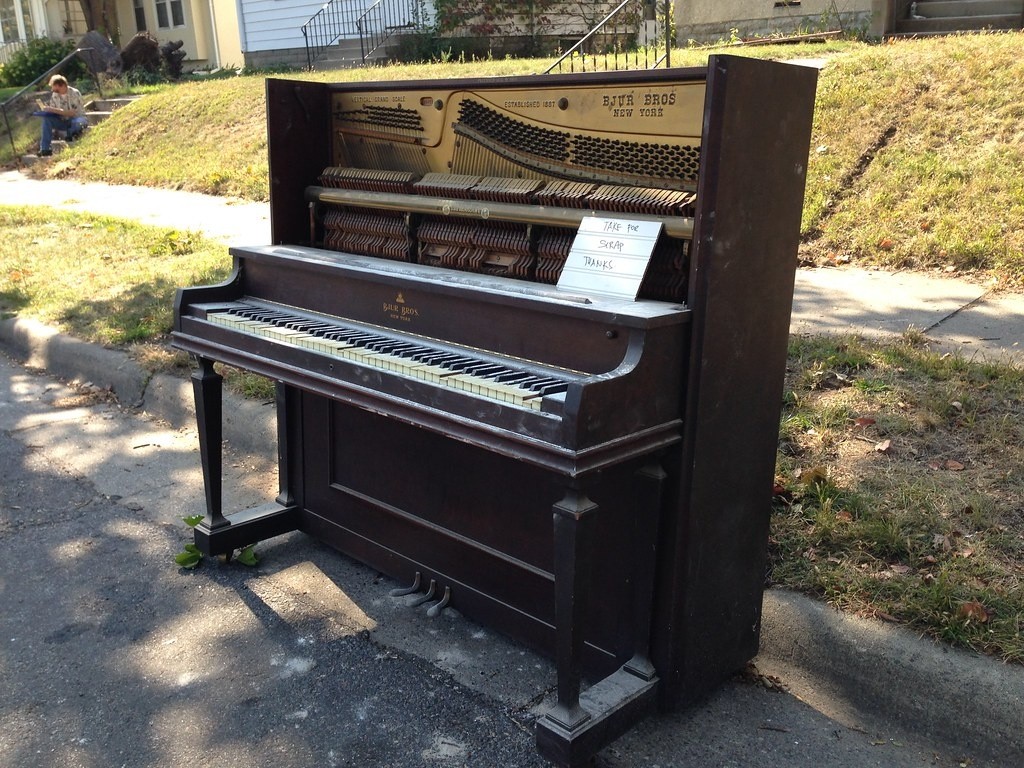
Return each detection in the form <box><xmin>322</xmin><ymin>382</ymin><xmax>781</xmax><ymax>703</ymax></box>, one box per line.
<box><xmin>169</xmin><ymin>53</ymin><xmax>819</xmax><ymax>768</ymax></box>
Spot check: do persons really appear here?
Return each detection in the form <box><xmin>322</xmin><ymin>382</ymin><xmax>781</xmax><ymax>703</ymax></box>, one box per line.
<box><xmin>33</xmin><ymin>74</ymin><xmax>88</xmax><ymax>157</ymax></box>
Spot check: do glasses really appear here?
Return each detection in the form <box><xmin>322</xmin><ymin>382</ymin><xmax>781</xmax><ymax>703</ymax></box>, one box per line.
<box><xmin>51</xmin><ymin>88</ymin><xmax>60</xmax><ymax>94</ymax></box>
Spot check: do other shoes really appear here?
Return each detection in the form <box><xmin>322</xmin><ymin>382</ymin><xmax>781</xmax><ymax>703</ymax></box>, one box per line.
<box><xmin>36</xmin><ymin>149</ymin><xmax>53</xmax><ymax>157</ymax></box>
